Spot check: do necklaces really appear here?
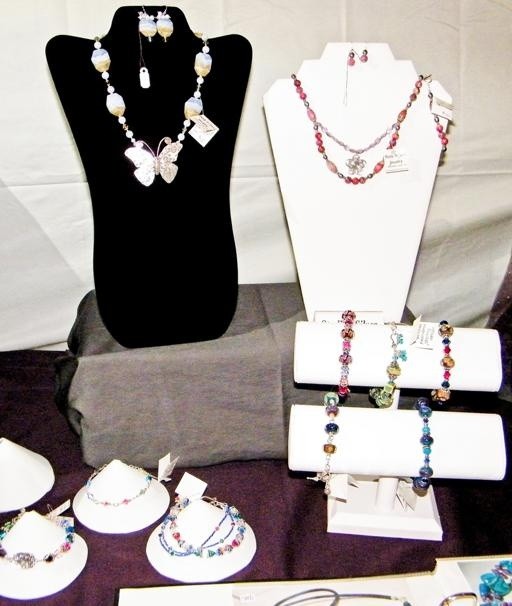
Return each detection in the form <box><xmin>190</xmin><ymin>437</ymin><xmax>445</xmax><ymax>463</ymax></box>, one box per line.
<box><xmin>287</xmin><ymin>42</ymin><xmax>426</xmax><ymax>186</ymax></box>
<box><xmin>91</xmin><ymin>1</ymin><xmax>216</xmax><ymax>186</ymax></box>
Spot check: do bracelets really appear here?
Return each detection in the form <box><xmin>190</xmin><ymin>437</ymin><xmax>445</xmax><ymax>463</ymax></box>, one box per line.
<box><xmin>428</xmin><ymin>78</ymin><xmax>456</xmax><ymax>152</ymax></box>
<box><xmin>413</xmin><ymin>396</ymin><xmax>437</xmax><ymax>497</ymax></box>
<box><xmin>85</xmin><ymin>463</ymin><xmax>154</xmax><ymax>506</ymax></box>
<box><xmin>338</xmin><ymin>309</ymin><xmax>356</xmax><ymax>398</ymax></box>
<box><xmin>0</xmin><ymin>510</ymin><xmax>74</xmax><ymax>572</ymax></box>
<box><xmin>430</xmin><ymin>319</ymin><xmax>455</xmax><ymax>405</ymax></box>
<box><xmin>319</xmin><ymin>391</ymin><xmax>340</xmax><ymax>482</ymax></box>
<box><xmin>369</xmin><ymin>320</ymin><xmax>409</xmax><ymax>408</ymax></box>
<box><xmin>159</xmin><ymin>496</ymin><xmax>247</xmax><ymax>558</ymax></box>
